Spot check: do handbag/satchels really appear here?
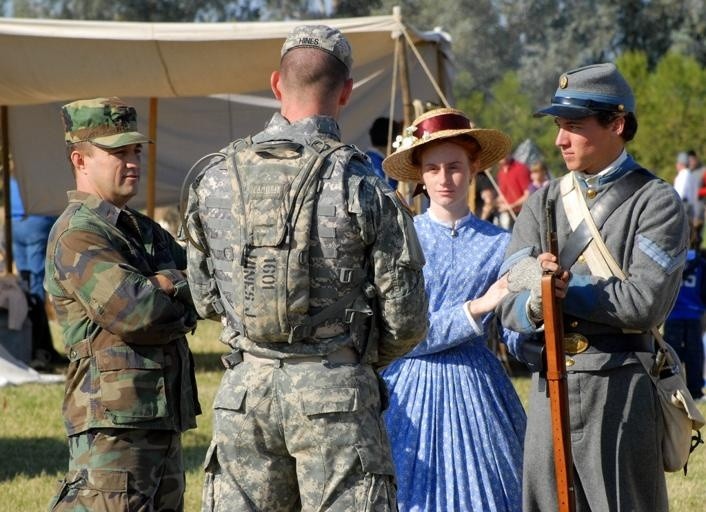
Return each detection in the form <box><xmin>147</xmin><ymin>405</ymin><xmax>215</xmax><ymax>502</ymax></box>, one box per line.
<box><xmin>656</xmin><ymin>374</ymin><xmax>704</xmax><ymax>471</ymax></box>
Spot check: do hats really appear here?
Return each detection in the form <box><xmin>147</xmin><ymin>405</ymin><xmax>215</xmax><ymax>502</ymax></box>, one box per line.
<box><xmin>280</xmin><ymin>24</ymin><xmax>352</xmax><ymax>78</ymax></box>
<box><xmin>60</xmin><ymin>96</ymin><xmax>153</xmax><ymax>149</ymax></box>
<box><xmin>533</xmin><ymin>63</ymin><xmax>634</xmax><ymax>119</ymax></box>
<box><xmin>381</xmin><ymin>108</ymin><xmax>511</xmax><ymax>183</ymax></box>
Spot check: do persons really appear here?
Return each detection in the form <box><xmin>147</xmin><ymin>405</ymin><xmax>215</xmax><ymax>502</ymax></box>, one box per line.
<box><xmin>494</xmin><ymin>154</ymin><xmax>528</xmax><ymax>232</ymax></box>
<box><xmin>185</xmin><ymin>24</ymin><xmax>431</xmax><ymax>512</ymax></box>
<box><xmin>476</xmin><ymin>171</ymin><xmax>496</xmax><ymax>223</ymax></box>
<box><xmin>10</xmin><ymin>175</ymin><xmax>70</xmax><ymax>372</ymax></box>
<box><xmin>375</xmin><ymin>108</ymin><xmax>529</xmax><ymax>512</ymax></box>
<box><xmin>495</xmin><ymin>63</ymin><xmax>692</xmax><ymax>512</ymax></box>
<box><xmin>44</xmin><ymin>95</ymin><xmax>199</xmax><ymax>512</ymax></box>
<box><xmin>529</xmin><ymin>163</ymin><xmax>550</xmax><ymax>194</ymax></box>
<box><xmin>674</xmin><ymin>152</ymin><xmax>692</xmax><ymax>202</ymax></box>
<box><xmin>689</xmin><ymin>151</ymin><xmax>706</xmax><ymax>214</ymax></box>
<box><xmin>366</xmin><ymin>117</ymin><xmax>403</xmax><ymax>192</ymax></box>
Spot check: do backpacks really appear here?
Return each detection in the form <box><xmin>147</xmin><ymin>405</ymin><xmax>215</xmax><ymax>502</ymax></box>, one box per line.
<box><xmin>218</xmin><ymin>135</ymin><xmax>360</xmax><ymax>343</ymax></box>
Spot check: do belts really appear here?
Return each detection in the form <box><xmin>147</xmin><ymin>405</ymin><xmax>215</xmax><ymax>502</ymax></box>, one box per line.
<box><xmin>237</xmin><ymin>348</ymin><xmax>356</xmax><ymax>365</ymax></box>
<box><xmin>559</xmin><ymin>332</ymin><xmax>655</xmax><ymax>355</ymax></box>
